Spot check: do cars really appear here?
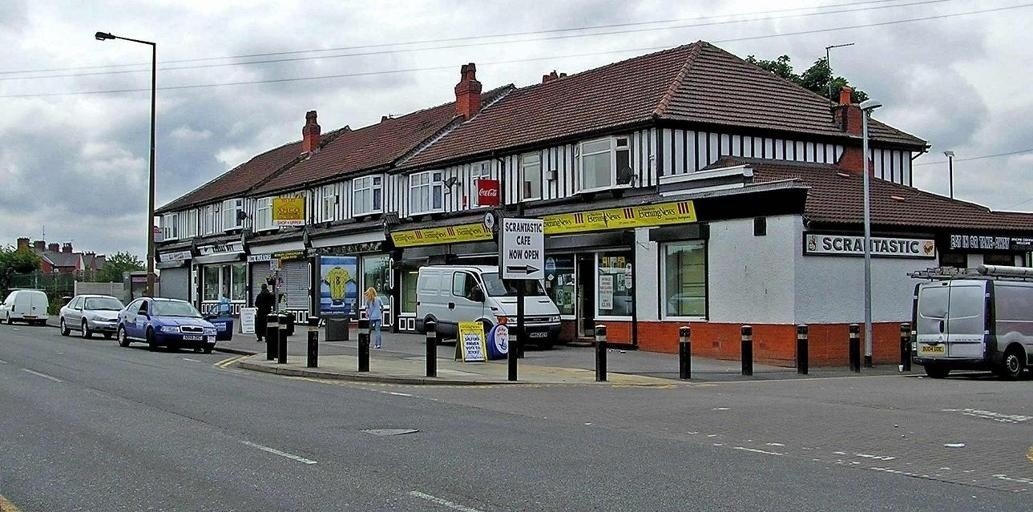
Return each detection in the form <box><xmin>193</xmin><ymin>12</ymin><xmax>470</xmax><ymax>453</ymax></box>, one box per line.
<box><xmin>115</xmin><ymin>296</ymin><xmax>235</xmax><ymax>353</ymax></box>
<box><xmin>56</xmin><ymin>293</ymin><xmax>123</xmax><ymax>339</ymax></box>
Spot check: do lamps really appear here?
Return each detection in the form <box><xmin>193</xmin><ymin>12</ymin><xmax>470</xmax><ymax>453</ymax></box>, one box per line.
<box><xmin>616</xmin><ymin>166</ymin><xmax>640</xmax><ymax>192</ymax></box>
<box><xmin>441</xmin><ymin>174</ymin><xmax>460</xmax><ymax>189</ymax></box>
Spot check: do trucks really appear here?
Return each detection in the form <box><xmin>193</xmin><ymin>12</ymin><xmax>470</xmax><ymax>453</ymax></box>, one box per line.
<box><xmin>906</xmin><ymin>262</ymin><xmax>1033</xmax><ymax>379</ymax></box>
<box><xmin>413</xmin><ymin>263</ymin><xmax>565</xmax><ymax>352</ymax></box>
<box><xmin>544</xmin><ymin>266</ymin><xmax>680</xmax><ymax>318</ymax></box>
<box><xmin>0</xmin><ymin>291</ymin><xmax>50</xmax><ymax>328</ymax></box>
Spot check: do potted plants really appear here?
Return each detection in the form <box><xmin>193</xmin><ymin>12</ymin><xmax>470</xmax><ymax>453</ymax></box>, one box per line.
<box><xmin>264</xmin><ymin>270</ymin><xmax>279</xmax><ymax>286</ymax></box>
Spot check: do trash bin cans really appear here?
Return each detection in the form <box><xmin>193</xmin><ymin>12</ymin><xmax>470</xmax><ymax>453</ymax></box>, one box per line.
<box><xmin>325</xmin><ymin>316</ymin><xmax>349</xmax><ymax>341</ymax></box>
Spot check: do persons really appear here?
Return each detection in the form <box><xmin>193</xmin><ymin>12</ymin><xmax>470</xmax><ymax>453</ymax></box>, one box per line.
<box><xmin>365</xmin><ymin>287</ymin><xmax>384</xmax><ymax>349</ymax></box>
<box><xmin>254</xmin><ymin>283</ymin><xmax>276</xmax><ymax>342</ymax></box>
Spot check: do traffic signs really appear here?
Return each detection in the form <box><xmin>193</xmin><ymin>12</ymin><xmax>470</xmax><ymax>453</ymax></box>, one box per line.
<box><xmin>499</xmin><ymin>216</ymin><xmax>546</xmax><ymax>283</ymax></box>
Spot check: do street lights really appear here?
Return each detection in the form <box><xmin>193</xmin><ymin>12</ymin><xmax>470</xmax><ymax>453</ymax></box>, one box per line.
<box><xmin>942</xmin><ymin>149</ymin><xmax>958</xmax><ymax>199</ymax></box>
<box><xmin>859</xmin><ymin>97</ymin><xmax>882</xmax><ymax>371</ymax></box>
<box><xmin>91</xmin><ymin>30</ymin><xmax>161</xmax><ymax>296</ymax></box>
